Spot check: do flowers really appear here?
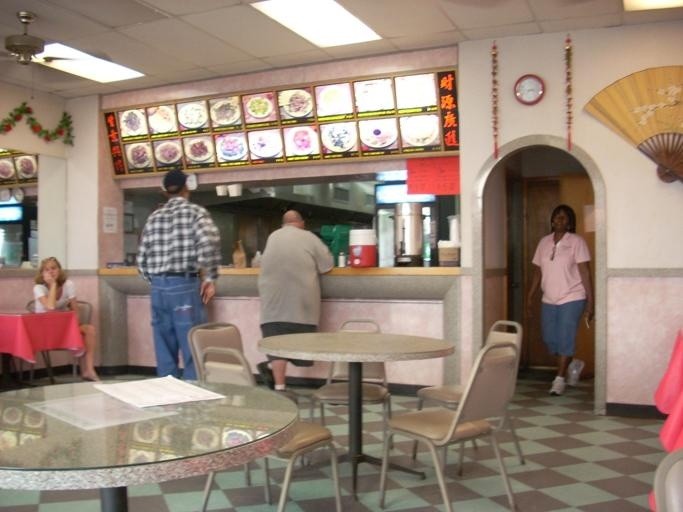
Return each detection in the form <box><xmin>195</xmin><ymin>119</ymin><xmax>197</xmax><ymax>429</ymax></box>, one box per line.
<box><xmin>0</xmin><ymin>101</ymin><xmax>75</xmax><ymax>146</ymax></box>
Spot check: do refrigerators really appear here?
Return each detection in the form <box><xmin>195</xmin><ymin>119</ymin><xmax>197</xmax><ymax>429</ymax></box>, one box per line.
<box><xmin>374</xmin><ymin>182</ymin><xmax>456</xmax><ymax>268</ymax></box>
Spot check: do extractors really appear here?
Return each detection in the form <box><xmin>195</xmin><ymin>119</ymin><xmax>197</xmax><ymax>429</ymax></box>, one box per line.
<box><xmin>194</xmin><ymin>179</ymin><xmax>373</xmax><ymax>222</ymax></box>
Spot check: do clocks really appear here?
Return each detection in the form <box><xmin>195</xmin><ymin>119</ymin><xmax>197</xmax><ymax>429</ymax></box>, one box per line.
<box><xmin>514</xmin><ymin>74</ymin><xmax>545</xmax><ymax>104</ymax></box>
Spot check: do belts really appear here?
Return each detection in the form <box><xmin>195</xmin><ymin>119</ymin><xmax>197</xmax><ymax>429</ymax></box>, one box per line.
<box><xmin>154</xmin><ymin>270</ymin><xmax>200</xmax><ymax>278</ymax></box>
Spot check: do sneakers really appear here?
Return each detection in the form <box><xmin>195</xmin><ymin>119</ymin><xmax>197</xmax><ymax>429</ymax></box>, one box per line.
<box><xmin>566</xmin><ymin>358</ymin><xmax>586</xmax><ymax>388</ymax></box>
<box><xmin>548</xmin><ymin>376</ymin><xmax>566</xmax><ymax>396</ymax></box>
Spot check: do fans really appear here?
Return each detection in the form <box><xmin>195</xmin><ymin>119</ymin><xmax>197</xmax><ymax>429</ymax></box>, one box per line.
<box><xmin>0</xmin><ymin>11</ymin><xmax>77</xmax><ymax>65</ymax></box>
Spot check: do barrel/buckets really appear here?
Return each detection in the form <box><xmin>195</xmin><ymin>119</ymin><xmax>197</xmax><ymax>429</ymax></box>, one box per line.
<box><xmin>394</xmin><ymin>201</ymin><xmax>424</xmax><ymax>258</ymax></box>
<box><xmin>346</xmin><ymin>228</ymin><xmax>378</xmax><ymax>269</ymax></box>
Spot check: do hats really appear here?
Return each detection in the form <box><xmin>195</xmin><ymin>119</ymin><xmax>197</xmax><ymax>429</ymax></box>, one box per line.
<box><xmin>256</xmin><ymin>361</ymin><xmax>276</xmax><ymax>389</ymax></box>
<box><xmin>163</xmin><ymin>170</ymin><xmax>189</xmax><ymax>194</ymax></box>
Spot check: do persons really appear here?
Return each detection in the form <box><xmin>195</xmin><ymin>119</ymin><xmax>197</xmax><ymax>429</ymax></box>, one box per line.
<box><xmin>136</xmin><ymin>169</ymin><xmax>223</xmax><ymax>383</ymax></box>
<box><xmin>256</xmin><ymin>210</ymin><xmax>335</xmax><ymax>392</ymax></box>
<box><xmin>526</xmin><ymin>204</ymin><xmax>594</xmax><ymax>396</ymax></box>
<box><xmin>32</xmin><ymin>257</ymin><xmax>100</xmax><ymax>381</ymax></box>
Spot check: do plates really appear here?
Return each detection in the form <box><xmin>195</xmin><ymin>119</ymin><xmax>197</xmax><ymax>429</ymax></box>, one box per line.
<box><xmin>0</xmin><ymin>155</ymin><xmax>37</xmax><ymax>180</ymax></box>
<box><xmin>120</xmin><ymin>74</ymin><xmax>438</xmax><ymax>172</ymax></box>
<box><xmin>0</xmin><ymin>187</ymin><xmax>25</xmax><ymax>202</ymax></box>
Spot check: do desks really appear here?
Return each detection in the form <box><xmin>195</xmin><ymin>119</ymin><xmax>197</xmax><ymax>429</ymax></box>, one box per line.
<box><xmin>257</xmin><ymin>331</ymin><xmax>456</xmax><ymax>502</ymax></box>
<box><xmin>0</xmin><ymin>313</ymin><xmax>85</xmax><ymax>387</ymax></box>
<box><xmin>0</xmin><ymin>379</ymin><xmax>301</xmax><ymax>512</ymax></box>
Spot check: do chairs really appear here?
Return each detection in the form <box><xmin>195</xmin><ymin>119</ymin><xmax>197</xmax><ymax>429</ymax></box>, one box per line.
<box><xmin>654</xmin><ymin>449</ymin><xmax>683</xmax><ymax>512</ymax></box>
<box><xmin>379</xmin><ymin>342</ymin><xmax>519</xmax><ymax>511</ymax></box>
<box><xmin>198</xmin><ymin>346</ymin><xmax>343</xmax><ymax>512</ymax></box>
<box><xmin>411</xmin><ymin>320</ymin><xmax>525</xmax><ymax>467</ymax></box>
<box><xmin>67</xmin><ymin>301</ymin><xmax>92</xmax><ymax>377</ymax></box>
<box><xmin>308</xmin><ymin>320</ymin><xmax>389</xmax><ymax>451</ymax></box>
<box><xmin>185</xmin><ymin>323</ymin><xmax>245</xmax><ymax>378</ymax></box>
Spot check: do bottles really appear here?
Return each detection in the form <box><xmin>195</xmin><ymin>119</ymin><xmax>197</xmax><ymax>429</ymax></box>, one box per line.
<box><xmin>31</xmin><ymin>255</ymin><xmax>38</xmax><ymax>268</ymax></box>
<box><xmin>250</xmin><ymin>249</ymin><xmax>263</xmax><ymax>270</ymax></box>
<box><xmin>337</xmin><ymin>252</ymin><xmax>346</xmax><ymax>268</ymax></box>
